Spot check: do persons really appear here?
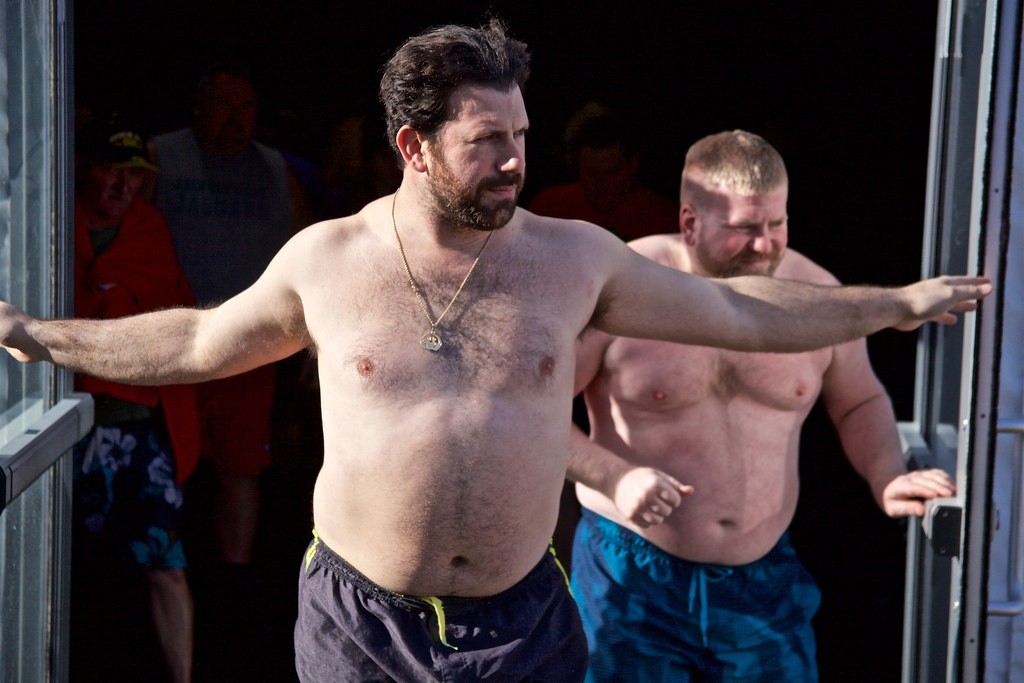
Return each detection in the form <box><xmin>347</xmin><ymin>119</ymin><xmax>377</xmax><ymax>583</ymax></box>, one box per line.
<box><xmin>141</xmin><ymin>64</ymin><xmax>317</xmax><ymax>630</ymax></box>
<box><xmin>1</xmin><ymin>13</ymin><xmax>996</xmax><ymax>683</ymax></box>
<box><xmin>63</xmin><ymin>117</ymin><xmax>211</xmax><ymax>680</ymax></box>
<box><xmin>554</xmin><ymin>126</ymin><xmax>960</xmax><ymax>683</ymax></box>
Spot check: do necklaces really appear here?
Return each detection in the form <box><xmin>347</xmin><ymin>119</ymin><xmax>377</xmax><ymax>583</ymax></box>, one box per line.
<box><xmin>391</xmin><ymin>185</ymin><xmax>499</xmax><ymax>354</ymax></box>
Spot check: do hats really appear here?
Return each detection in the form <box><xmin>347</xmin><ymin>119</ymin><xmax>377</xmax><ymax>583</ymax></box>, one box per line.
<box><xmin>82</xmin><ymin>108</ymin><xmax>161</xmax><ymax>175</ymax></box>
